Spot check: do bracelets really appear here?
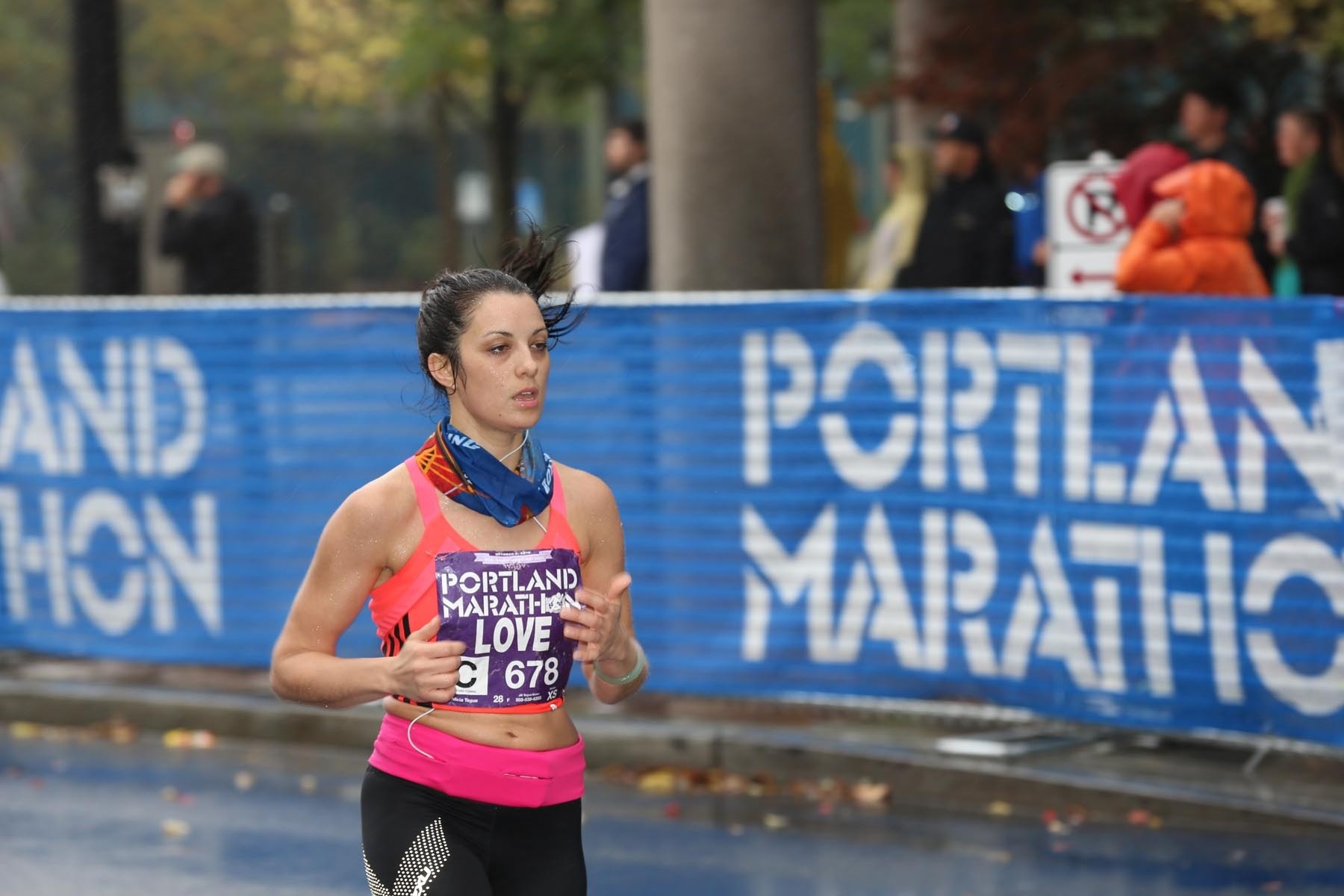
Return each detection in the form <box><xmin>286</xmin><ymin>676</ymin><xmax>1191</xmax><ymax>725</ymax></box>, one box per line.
<box><xmin>592</xmin><ymin>639</ymin><xmax>645</xmax><ymax>686</ymax></box>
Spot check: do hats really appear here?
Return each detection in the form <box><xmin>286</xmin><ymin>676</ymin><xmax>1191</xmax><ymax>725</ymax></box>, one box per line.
<box><xmin>925</xmin><ymin>114</ymin><xmax>985</xmax><ymax>144</ymax></box>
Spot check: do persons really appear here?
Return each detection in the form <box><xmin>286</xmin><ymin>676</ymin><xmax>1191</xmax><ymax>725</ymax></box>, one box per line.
<box><xmin>153</xmin><ymin>140</ymin><xmax>266</xmax><ymax>296</ymax></box>
<box><xmin>599</xmin><ymin>117</ymin><xmax>652</xmax><ymax>291</ymax></box>
<box><xmin>858</xmin><ymin>79</ymin><xmax>1344</xmax><ymax>300</ymax></box>
<box><xmin>269</xmin><ymin>235</ymin><xmax>652</xmax><ymax>896</ymax></box>
<box><xmin>77</xmin><ymin>147</ymin><xmax>147</xmax><ymax>296</ymax></box>
